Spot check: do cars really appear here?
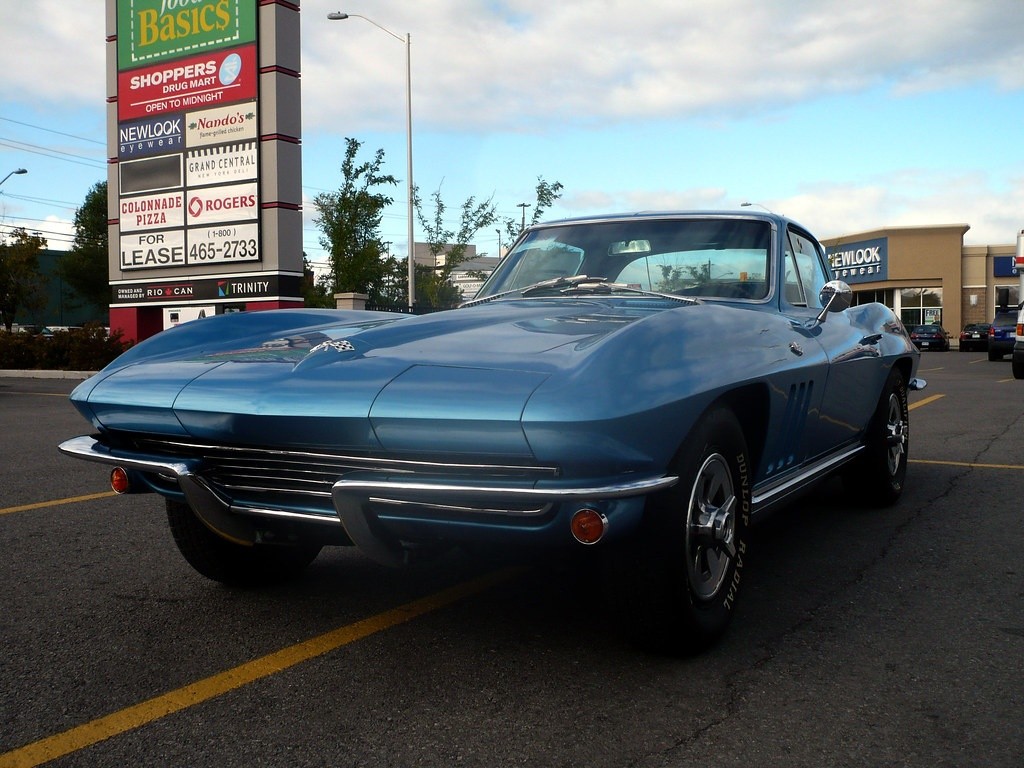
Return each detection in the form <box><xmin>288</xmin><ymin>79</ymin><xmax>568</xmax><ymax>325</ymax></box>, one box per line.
<box><xmin>910</xmin><ymin>323</ymin><xmax>954</xmax><ymax>352</ymax></box>
<box><xmin>958</xmin><ymin>322</ymin><xmax>992</xmax><ymax>352</ymax></box>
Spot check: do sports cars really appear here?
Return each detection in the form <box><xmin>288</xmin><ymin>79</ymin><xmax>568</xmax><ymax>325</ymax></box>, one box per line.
<box><xmin>57</xmin><ymin>207</ymin><xmax>930</xmax><ymax>654</ymax></box>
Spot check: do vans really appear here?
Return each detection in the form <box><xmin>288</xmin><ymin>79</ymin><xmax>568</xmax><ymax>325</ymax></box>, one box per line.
<box><xmin>998</xmin><ymin>229</ymin><xmax>1024</xmax><ymax>380</ymax></box>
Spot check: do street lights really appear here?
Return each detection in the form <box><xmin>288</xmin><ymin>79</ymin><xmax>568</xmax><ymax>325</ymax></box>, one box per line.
<box><xmin>516</xmin><ymin>203</ymin><xmax>533</xmax><ymax>230</ymax></box>
<box><xmin>384</xmin><ymin>241</ymin><xmax>394</xmax><ymax>298</ymax></box>
<box><xmin>495</xmin><ymin>229</ymin><xmax>501</xmax><ymax>262</ymax></box>
<box><xmin>325</xmin><ymin>10</ymin><xmax>417</xmax><ymax>314</ymax></box>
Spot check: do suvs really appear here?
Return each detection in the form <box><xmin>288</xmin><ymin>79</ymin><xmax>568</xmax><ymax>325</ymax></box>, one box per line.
<box><xmin>987</xmin><ymin>310</ymin><xmax>1019</xmax><ymax>362</ymax></box>
